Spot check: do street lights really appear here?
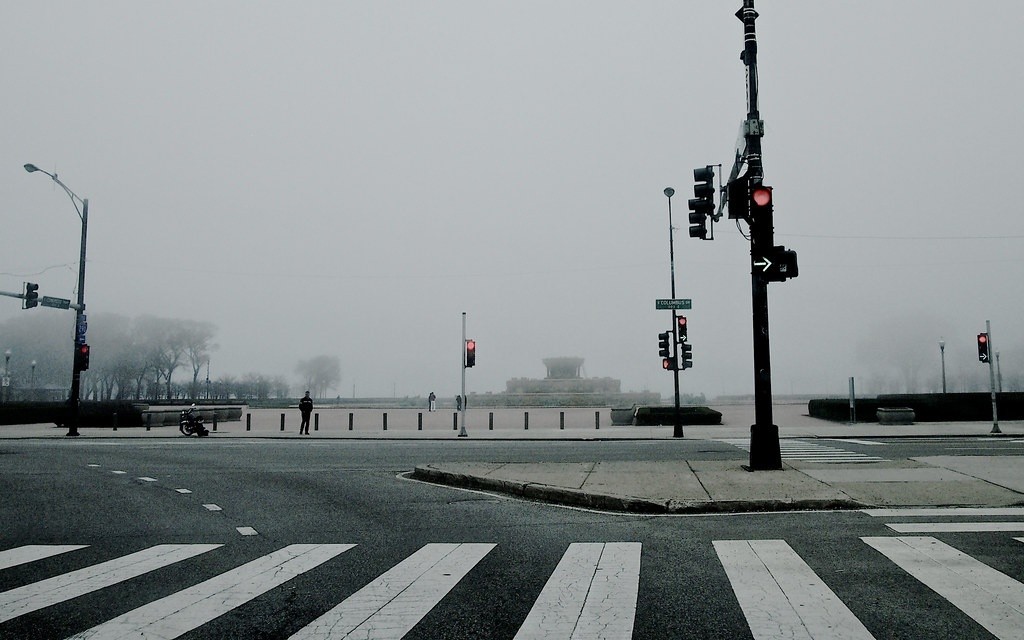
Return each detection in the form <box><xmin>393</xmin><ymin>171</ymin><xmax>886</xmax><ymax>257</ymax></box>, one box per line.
<box><xmin>663</xmin><ymin>186</ymin><xmax>684</xmax><ymax>437</ymax></box>
<box><xmin>938</xmin><ymin>338</ymin><xmax>946</xmax><ymax>394</ymax></box>
<box><xmin>22</xmin><ymin>162</ymin><xmax>91</xmax><ymax>436</ymax></box>
<box><xmin>30</xmin><ymin>359</ymin><xmax>36</xmax><ymax>400</ymax></box>
<box><xmin>3</xmin><ymin>348</ymin><xmax>12</xmax><ymax>404</ymax></box>
<box><xmin>994</xmin><ymin>350</ymin><xmax>1003</xmax><ymax>392</ymax></box>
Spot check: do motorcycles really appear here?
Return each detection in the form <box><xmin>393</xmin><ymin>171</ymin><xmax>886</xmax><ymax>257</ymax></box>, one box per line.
<box><xmin>179</xmin><ymin>403</ymin><xmax>205</xmax><ymax>437</ymax></box>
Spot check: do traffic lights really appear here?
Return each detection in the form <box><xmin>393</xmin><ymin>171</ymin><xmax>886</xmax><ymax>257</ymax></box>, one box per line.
<box><xmin>693</xmin><ymin>165</ymin><xmax>715</xmax><ymax>216</ymax></box>
<box><xmin>80</xmin><ymin>344</ymin><xmax>91</xmax><ymax>370</ymax></box>
<box><xmin>466</xmin><ymin>341</ymin><xmax>476</xmax><ymax>366</ymax></box>
<box><xmin>751</xmin><ymin>185</ymin><xmax>772</xmax><ymax>208</ymax></box>
<box><xmin>977</xmin><ymin>334</ymin><xmax>988</xmax><ymax>362</ymax></box>
<box><xmin>678</xmin><ymin>316</ymin><xmax>688</xmax><ymax>342</ymax></box>
<box><xmin>25</xmin><ymin>282</ymin><xmax>39</xmax><ymax>309</ymax></box>
<box><xmin>681</xmin><ymin>344</ymin><xmax>692</xmax><ymax>369</ymax></box>
<box><xmin>686</xmin><ymin>196</ymin><xmax>708</xmax><ymax>241</ymax></box>
<box><xmin>658</xmin><ymin>333</ymin><xmax>670</xmax><ymax>369</ymax></box>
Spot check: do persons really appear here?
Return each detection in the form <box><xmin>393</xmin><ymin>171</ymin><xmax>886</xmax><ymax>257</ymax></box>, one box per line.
<box><xmin>428</xmin><ymin>392</ymin><xmax>467</xmax><ymax>412</ymax></box>
<box><xmin>299</xmin><ymin>391</ymin><xmax>313</xmax><ymax>435</ymax></box>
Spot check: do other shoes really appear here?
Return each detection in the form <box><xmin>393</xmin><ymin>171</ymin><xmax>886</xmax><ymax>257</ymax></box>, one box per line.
<box><xmin>298</xmin><ymin>431</ymin><xmax>311</xmax><ymax>435</ymax></box>
